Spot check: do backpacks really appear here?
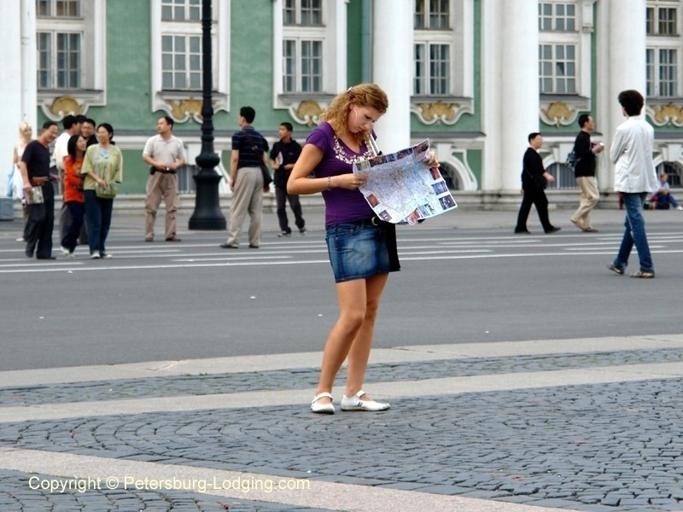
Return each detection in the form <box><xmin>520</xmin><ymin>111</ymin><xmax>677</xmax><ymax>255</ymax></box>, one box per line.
<box><xmin>565</xmin><ymin>138</ymin><xmax>591</xmax><ymax>172</ymax></box>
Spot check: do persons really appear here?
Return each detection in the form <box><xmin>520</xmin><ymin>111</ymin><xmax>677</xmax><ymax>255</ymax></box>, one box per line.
<box><xmin>82</xmin><ymin>119</ymin><xmax>115</xmax><ymax>159</ymax></box>
<box><xmin>269</xmin><ymin>122</ymin><xmax>307</xmax><ymax>236</ymax></box>
<box><xmin>10</xmin><ymin>121</ymin><xmax>35</xmax><ymax>242</ymax></box>
<box><xmin>287</xmin><ymin>82</ymin><xmax>440</xmax><ymax>415</ymax></box>
<box><xmin>59</xmin><ymin>135</ymin><xmax>86</xmax><ymax>256</ymax></box>
<box><xmin>607</xmin><ymin>91</ymin><xmax>657</xmax><ymax>278</ymax></box>
<box><xmin>571</xmin><ymin>112</ymin><xmax>605</xmax><ymax>233</ymax></box>
<box><xmin>82</xmin><ymin>123</ymin><xmax>123</xmax><ymax>258</ymax></box>
<box><xmin>650</xmin><ymin>174</ymin><xmax>673</xmax><ymax>197</ymax></box>
<box><xmin>221</xmin><ymin>106</ymin><xmax>268</xmax><ymax>249</ymax></box>
<box><xmin>141</xmin><ymin>117</ymin><xmax>185</xmax><ymax>242</ymax></box>
<box><xmin>18</xmin><ymin>122</ymin><xmax>58</xmax><ymax>260</ymax></box>
<box><xmin>515</xmin><ymin>133</ymin><xmax>561</xmax><ymax>234</ymax></box>
<box><xmin>51</xmin><ymin>114</ymin><xmax>80</xmax><ymax>252</ymax></box>
<box><xmin>643</xmin><ymin>174</ymin><xmax>682</xmax><ymax>211</ymax></box>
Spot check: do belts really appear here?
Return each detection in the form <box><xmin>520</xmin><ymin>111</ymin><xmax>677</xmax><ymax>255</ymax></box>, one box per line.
<box><xmin>152</xmin><ymin>166</ymin><xmax>176</xmax><ymax>173</ymax></box>
<box><xmin>347</xmin><ymin>215</ymin><xmax>385</xmax><ymax>228</ymax></box>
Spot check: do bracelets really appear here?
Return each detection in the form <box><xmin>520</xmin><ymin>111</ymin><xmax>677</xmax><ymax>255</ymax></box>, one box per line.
<box><xmin>327</xmin><ymin>176</ymin><xmax>330</xmax><ymax>191</ymax></box>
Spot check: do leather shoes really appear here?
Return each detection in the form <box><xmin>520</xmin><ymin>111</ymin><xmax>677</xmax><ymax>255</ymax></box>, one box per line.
<box><xmin>167</xmin><ymin>236</ymin><xmax>181</xmax><ymax>242</ymax></box>
<box><xmin>91</xmin><ymin>250</ymin><xmax>100</xmax><ymax>258</ymax></box>
<box><xmin>581</xmin><ymin>227</ymin><xmax>600</xmax><ymax>232</ymax></box>
<box><xmin>340</xmin><ymin>390</ymin><xmax>391</xmax><ymax>412</ymax></box>
<box><xmin>102</xmin><ymin>253</ymin><xmax>112</xmax><ymax>259</ymax></box>
<box><xmin>310</xmin><ymin>392</ymin><xmax>336</xmax><ymax>415</ymax></box>
<box><xmin>569</xmin><ymin>218</ymin><xmax>584</xmax><ymax>230</ymax></box>
<box><xmin>219</xmin><ymin>242</ymin><xmax>238</xmax><ymax>249</ymax></box>
<box><xmin>38</xmin><ymin>255</ymin><xmax>56</xmax><ymax>260</ymax></box>
<box><xmin>514</xmin><ymin>230</ymin><xmax>530</xmax><ymax>235</ymax></box>
<box><xmin>545</xmin><ymin>226</ymin><xmax>560</xmax><ymax>234</ymax></box>
<box><xmin>249</xmin><ymin>243</ymin><xmax>259</xmax><ymax>249</ymax></box>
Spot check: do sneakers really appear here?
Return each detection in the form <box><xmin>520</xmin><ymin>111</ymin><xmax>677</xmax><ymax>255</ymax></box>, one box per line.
<box><xmin>607</xmin><ymin>263</ymin><xmax>623</xmax><ymax>275</ymax></box>
<box><xmin>630</xmin><ymin>269</ymin><xmax>655</xmax><ymax>279</ymax></box>
<box><xmin>278</xmin><ymin>230</ymin><xmax>289</xmax><ymax>237</ymax></box>
<box><xmin>299</xmin><ymin>227</ymin><xmax>306</xmax><ymax>234</ymax></box>
